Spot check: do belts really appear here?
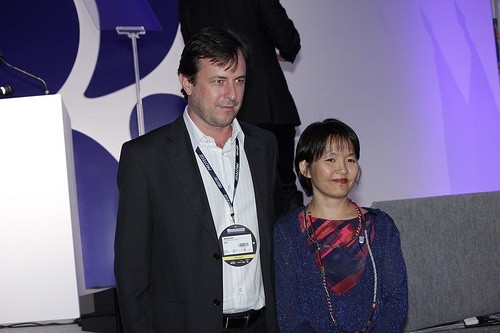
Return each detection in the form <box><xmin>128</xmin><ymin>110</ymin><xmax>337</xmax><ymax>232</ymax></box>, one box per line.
<box><xmin>224</xmin><ymin>306</ymin><xmax>266</xmax><ymax>330</ymax></box>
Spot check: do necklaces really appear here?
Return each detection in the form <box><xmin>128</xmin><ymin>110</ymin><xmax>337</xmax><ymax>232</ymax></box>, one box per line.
<box><xmin>308</xmin><ymin>201</ymin><xmax>379</xmax><ymax>333</ymax></box>
<box><xmin>304</xmin><ymin>198</ymin><xmax>363</xmax><ymax>249</ymax></box>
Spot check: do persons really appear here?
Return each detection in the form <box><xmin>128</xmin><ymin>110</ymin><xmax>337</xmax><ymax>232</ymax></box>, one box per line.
<box><xmin>273</xmin><ymin>117</ymin><xmax>410</xmax><ymax>333</ymax></box>
<box><xmin>173</xmin><ymin>0</ymin><xmax>302</xmax><ymax>221</ymax></box>
<box><xmin>114</xmin><ymin>26</ymin><xmax>307</xmax><ymax>333</ymax></box>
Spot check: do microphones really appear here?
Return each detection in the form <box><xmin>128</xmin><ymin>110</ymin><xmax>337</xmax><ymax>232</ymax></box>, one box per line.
<box><xmin>0</xmin><ymin>58</ymin><xmax>49</xmax><ymax>95</ymax></box>
